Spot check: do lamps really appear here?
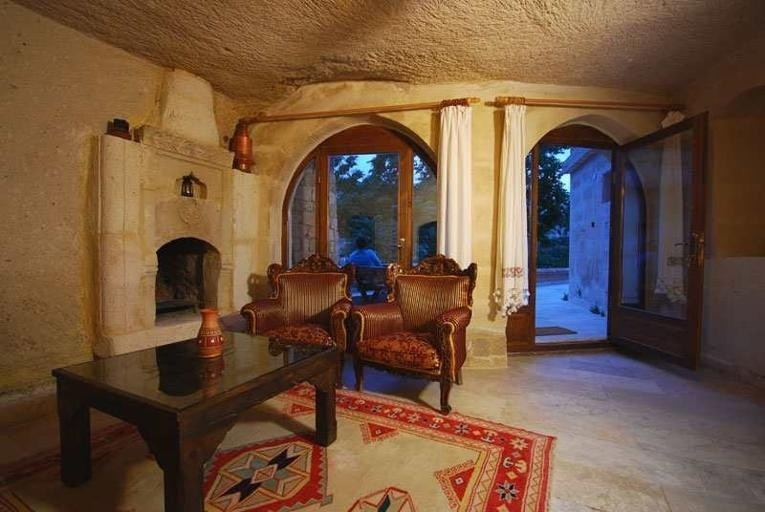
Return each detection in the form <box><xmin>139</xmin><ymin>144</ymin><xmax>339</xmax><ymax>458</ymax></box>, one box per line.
<box><xmin>241</xmin><ymin>255</ymin><xmax>478</xmax><ymax>416</ymax></box>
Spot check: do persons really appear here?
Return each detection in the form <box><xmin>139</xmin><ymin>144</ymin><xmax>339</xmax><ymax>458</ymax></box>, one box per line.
<box><xmin>349</xmin><ymin>236</ymin><xmax>383</xmax><ymax>303</ymax></box>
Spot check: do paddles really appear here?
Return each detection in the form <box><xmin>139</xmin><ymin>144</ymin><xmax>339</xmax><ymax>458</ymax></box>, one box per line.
<box><xmin>536</xmin><ymin>326</ymin><xmax>576</xmax><ymax>337</ymax></box>
<box><xmin>1</xmin><ymin>382</ymin><xmax>556</xmax><ymax>512</ymax></box>
